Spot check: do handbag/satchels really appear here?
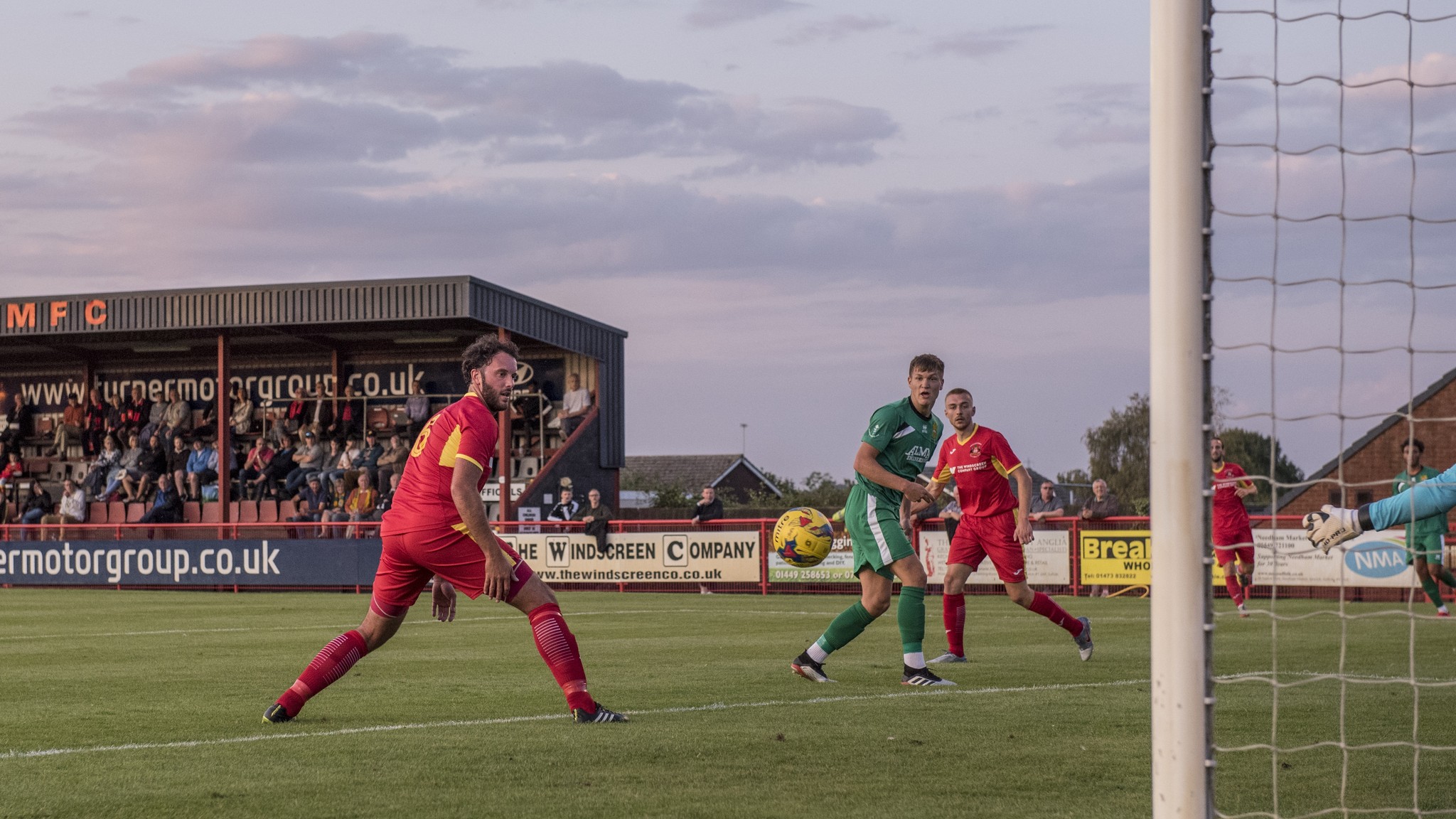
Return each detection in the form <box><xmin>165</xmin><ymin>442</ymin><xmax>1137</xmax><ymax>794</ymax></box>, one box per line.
<box><xmin>107</xmin><ymin>492</ymin><xmax>121</xmax><ymax>502</ymax></box>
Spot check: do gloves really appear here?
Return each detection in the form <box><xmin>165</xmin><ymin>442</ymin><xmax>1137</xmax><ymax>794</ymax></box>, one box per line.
<box><xmin>1302</xmin><ymin>505</ymin><xmax>1365</xmax><ymax>555</ymax></box>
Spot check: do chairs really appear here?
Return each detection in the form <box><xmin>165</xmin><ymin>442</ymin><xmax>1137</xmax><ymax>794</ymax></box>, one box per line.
<box><xmin>1</xmin><ymin>406</ymin><xmax>408</xmax><ymax>540</ymax></box>
<box><xmin>488</xmin><ymin>502</ymin><xmax>501</xmax><ymax>528</ymax></box>
<box><xmin>517</xmin><ymin>457</ymin><xmax>539</xmax><ymax>479</ymax></box>
<box><xmin>494</xmin><ymin>458</ymin><xmax>516</xmax><ymax>478</ymax></box>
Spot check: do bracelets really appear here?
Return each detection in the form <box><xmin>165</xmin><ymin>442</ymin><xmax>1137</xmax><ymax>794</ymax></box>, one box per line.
<box><xmin>949</xmin><ymin>510</ymin><xmax>951</xmax><ymax>514</ymax></box>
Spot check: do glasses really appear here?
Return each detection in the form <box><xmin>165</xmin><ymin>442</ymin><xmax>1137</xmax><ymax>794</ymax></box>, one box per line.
<box><xmin>1041</xmin><ymin>486</ymin><xmax>1053</xmax><ymax>490</ymax></box>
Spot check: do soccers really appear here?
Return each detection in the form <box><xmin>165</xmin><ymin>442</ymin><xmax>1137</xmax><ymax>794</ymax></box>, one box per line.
<box><xmin>773</xmin><ymin>506</ymin><xmax>833</xmax><ymax>569</ymax></box>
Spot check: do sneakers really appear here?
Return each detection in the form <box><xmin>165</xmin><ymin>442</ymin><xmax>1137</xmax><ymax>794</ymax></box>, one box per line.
<box><xmin>790</xmin><ymin>648</ymin><xmax>836</xmax><ymax>682</ymax></box>
<box><xmin>95</xmin><ymin>493</ymin><xmax>107</xmax><ymax>502</ymax></box>
<box><xmin>926</xmin><ymin>648</ymin><xmax>967</xmax><ymax>663</ymax></box>
<box><xmin>1238</xmin><ymin>604</ymin><xmax>1249</xmax><ymax>617</ymax></box>
<box><xmin>245</xmin><ymin>481</ymin><xmax>257</xmax><ymax>488</ymax></box>
<box><xmin>237</xmin><ymin>496</ymin><xmax>247</xmax><ymax>501</ymax></box>
<box><xmin>1073</xmin><ymin>617</ymin><xmax>1093</xmax><ymax>661</ymax></box>
<box><xmin>1235</xmin><ymin>565</ymin><xmax>1248</xmax><ymax>587</ymax></box>
<box><xmin>261</xmin><ymin>703</ymin><xmax>292</xmax><ymax>724</ymax></box>
<box><xmin>559</xmin><ymin>429</ymin><xmax>568</xmax><ymax>440</ymax></box>
<box><xmin>43</xmin><ymin>449</ymin><xmax>57</xmax><ymax>457</ymax></box>
<box><xmin>572</xmin><ymin>701</ymin><xmax>630</xmax><ymax>723</ymax></box>
<box><xmin>901</xmin><ymin>662</ymin><xmax>958</xmax><ymax>685</ymax></box>
<box><xmin>1437</xmin><ymin>612</ymin><xmax>1450</xmax><ymax>616</ymax></box>
<box><xmin>58</xmin><ymin>453</ymin><xmax>67</xmax><ymax>462</ymax></box>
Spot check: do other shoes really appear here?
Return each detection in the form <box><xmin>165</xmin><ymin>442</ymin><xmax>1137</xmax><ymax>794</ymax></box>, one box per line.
<box><xmin>81</xmin><ymin>456</ymin><xmax>92</xmax><ymax>462</ymax></box>
<box><xmin>123</xmin><ymin>497</ymin><xmax>141</xmax><ymax>503</ymax></box>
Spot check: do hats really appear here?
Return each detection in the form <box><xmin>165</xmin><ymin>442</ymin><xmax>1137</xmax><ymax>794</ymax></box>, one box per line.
<box><xmin>365</xmin><ymin>430</ymin><xmax>377</xmax><ymax>437</ymax></box>
<box><xmin>192</xmin><ymin>436</ymin><xmax>204</xmax><ymax>442</ymax></box>
<box><xmin>310</xmin><ymin>476</ymin><xmax>320</xmax><ymax>482</ymax></box>
<box><xmin>68</xmin><ymin>393</ymin><xmax>78</xmax><ymax>399</ymax></box>
<box><xmin>305</xmin><ymin>432</ymin><xmax>315</xmax><ymax>438</ymax></box>
<box><xmin>347</xmin><ymin>433</ymin><xmax>357</xmax><ymax>441</ymax></box>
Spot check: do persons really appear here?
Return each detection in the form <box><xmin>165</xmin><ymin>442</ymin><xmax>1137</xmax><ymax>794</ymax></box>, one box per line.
<box><xmin>1026</xmin><ymin>480</ymin><xmax>1065</xmax><ymax>594</ymax></box>
<box><xmin>910</xmin><ymin>499</ymin><xmax>942</xmax><ymax>593</ymax></box>
<box><xmin>557</xmin><ymin>374</ymin><xmax>593</xmax><ymax>441</ymax></box>
<box><xmin>1076</xmin><ymin>478</ymin><xmax>1118</xmax><ymax>599</ymax></box>
<box><xmin>547</xmin><ymin>488</ymin><xmax>580</xmax><ymax>591</ymax></box>
<box><xmin>939</xmin><ymin>485</ymin><xmax>964</xmax><ymax>522</ymax></box>
<box><xmin>0</xmin><ymin>452</ymin><xmax>22</xmax><ymax>490</ymax></box>
<box><xmin>39</xmin><ymin>479</ymin><xmax>87</xmax><ymax>541</ymax></box>
<box><xmin>392</xmin><ymin>380</ymin><xmax>430</xmax><ymax>448</ymax></box>
<box><xmin>12</xmin><ymin>478</ymin><xmax>52</xmax><ymax>541</ymax></box>
<box><xmin>908</xmin><ymin>388</ymin><xmax>1094</xmax><ymax>661</ymax></box>
<box><xmin>511</xmin><ymin>380</ymin><xmax>550</xmax><ymax>455</ymax></box>
<box><xmin>128</xmin><ymin>474</ymin><xmax>187</xmax><ymax>527</ymax></box>
<box><xmin>1209</xmin><ymin>437</ymin><xmax>1259</xmax><ymax>618</ymax></box>
<box><xmin>46</xmin><ymin>380</ymin><xmax>430</xmax><ymax>538</ymax></box>
<box><xmin>573</xmin><ymin>488</ymin><xmax>615</xmax><ymax>591</ymax></box>
<box><xmin>1302</xmin><ymin>461</ymin><xmax>1456</xmax><ymax>563</ymax></box>
<box><xmin>1389</xmin><ymin>437</ymin><xmax>1456</xmax><ymax>615</ymax></box>
<box><xmin>1</xmin><ymin>391</ymin><xmax>35</xmax><ymax>449</ymax></box>
<box><xmin>263</xmin><ymin>337</ymin><xmax>630</xmax><ymax>723</ymax></box>
<box><xmin>789</xmin><ymin>353</ymin><xmax>958</xmax><ymax>689</ymax></box>
<box><xmin>690</xmin><ymin>486</ymin><xmax>724</xmax><ymax>595</ymax></box>
